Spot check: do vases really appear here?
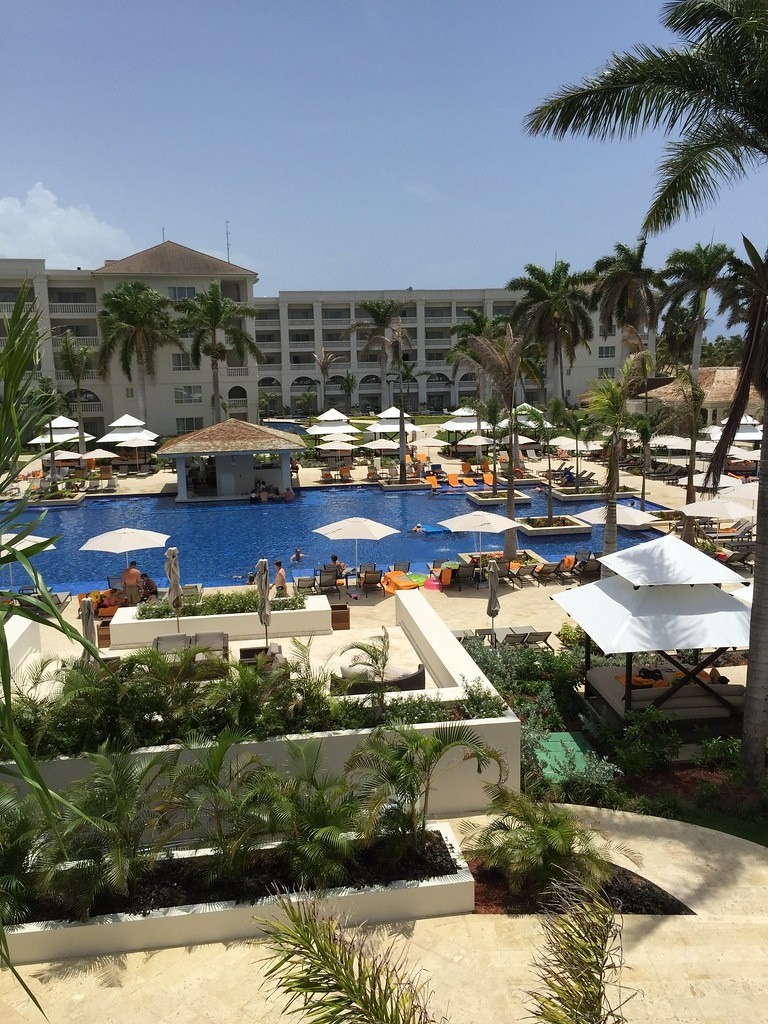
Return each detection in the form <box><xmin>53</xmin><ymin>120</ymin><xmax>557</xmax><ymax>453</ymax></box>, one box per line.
<box><xmin>352</xmin><ymin>412</ymin><xmax>362</xmax><ymax>417</ymax></box>
<box><xmin>418</xmin><ymin>410</ymin><xmax>430</xmax><ymax>414</ymax></box>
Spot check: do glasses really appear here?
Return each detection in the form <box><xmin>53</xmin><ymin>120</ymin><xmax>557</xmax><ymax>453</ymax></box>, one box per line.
<box><xmin>141</xmin><ymin>577</ymin><xmax>144</xmax><ymax>579</ymax></box>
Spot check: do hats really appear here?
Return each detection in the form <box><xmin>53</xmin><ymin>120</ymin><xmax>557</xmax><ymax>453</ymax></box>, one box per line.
<box><xmin>130</xmin><ymin>561</ymin><xmax>136</xmax><ymax>565</ymax></box>
<box><xmin>417</xmin><ymin>524</ymin><xmax>421</xmax><ymax>528</ymax></box>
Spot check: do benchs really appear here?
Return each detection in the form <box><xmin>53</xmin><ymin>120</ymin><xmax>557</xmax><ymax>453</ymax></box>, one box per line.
<box><xmin>330</xmin><ymin>662</ymin><xmax>425</xmax><ymax>697</ymax></box>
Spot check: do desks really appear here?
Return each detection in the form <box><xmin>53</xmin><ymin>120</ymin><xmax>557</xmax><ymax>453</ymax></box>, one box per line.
<box><xmin>426</xmin><ymin>471</ymin><xmax>434</xmax><ymax>475</ymax></box>
<box><xmin>379</xmin><ymin>473</ymin><xmax>388</xmax><ymax>478</ymax></box>
<box><xmin>335</xmin><ymin>474</ymin><xmax>341</xmax><ymax>482</ymax></box>
<box><xmin>475</xmin><ymin>629</ymin><xmax>496</xmax><ymax>650</ymax></box>
<box><xmin>345</xmin><ymin>571</ymin><xmax>361</xmax><ymax>589</ymax></box>
<box><xmin>475</xmin><ymin>469</ymin><xmax>482</xmax><ymax>474</ymax></box>
<box><xmin>19</xmin><ymin>586</ymin><xmax>37</xmax><ymax>594</ymax></box>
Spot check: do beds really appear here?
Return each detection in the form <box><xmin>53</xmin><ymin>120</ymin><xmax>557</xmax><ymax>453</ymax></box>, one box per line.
<box><xmin>448</xmin><ymin>430</ymin><xmax>489</xmax><ymax>459</ymax></box>
<box><xmin>111</xmin><ymin>442</ymin><xmax>151</xmax><ymax>470</ymax></box>
<box><xmin>374</xmin><ymin>432</ymin><xmax>414</xmax><ymax>458</ymax></box>
<box><xmin>584</xmin><ymin>632</ymin><xmax>744</xmax><ymax>740</ymax></box>
<box><xmin>38</xmin><ymin>443</ymin><xmax>80</xmax><ymax>472</ymax></box>
<box><xmin>314</xmin><ymin>434</ymin><xmax>354</xmax><ymax>462</ymax></box>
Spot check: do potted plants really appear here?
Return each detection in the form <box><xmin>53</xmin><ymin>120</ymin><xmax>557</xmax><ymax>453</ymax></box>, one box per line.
<box><xmin>0</xmin><ymin>626</ymin><xmax>522</xmax><ymax>846</ymax></box>
<box><xmin>0</xmin><ymin>711</ymin><xmax>507</xmax><ymax>965</ymax></box>
<box><xmin>282</xmin><ymin>405</ymin><xmax>292</xmax><ymax>418</ymax></box>
<box><xmin>109</xmin><ymin>595</ymin><xmax>332</xmax><ymax>645</ymax></box>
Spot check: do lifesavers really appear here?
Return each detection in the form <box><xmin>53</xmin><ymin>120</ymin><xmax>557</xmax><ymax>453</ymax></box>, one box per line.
<box><xmin>424</xmin><ymin>578</ymin><xmax>443</xmax><ymax>590</ymax></box>
<box><xmin>514</xmin><ymin>469</ymin><xmax>523</xmax><ymax>479</ymax></box>
<box><xmin>406</xmin><ymin>572</ymin><xmax>428</xmax><ymax>586</ymax></box>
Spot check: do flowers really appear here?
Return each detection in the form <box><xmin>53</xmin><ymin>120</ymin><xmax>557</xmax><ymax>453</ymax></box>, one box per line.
<box><xmin>351</xmin><ymin>403</ymin><xmax>360</xmax><ymax>414</ymax></box>
<box><xmin>418</xmin><ymin>402</ymin><xmax>428</xmax><ymax>411</ymax></box>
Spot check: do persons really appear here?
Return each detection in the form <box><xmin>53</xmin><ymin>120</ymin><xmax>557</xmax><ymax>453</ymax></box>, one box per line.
<box><xmin>140</xmin><ymin>573</ymin><xmax>158</xmax><ymax>601</ymax></box>
<box><xmin>560</xmin><ymin>468</ymin><xmax>573</xmax><ymax>486</ymax></box>
<box><xmin>290</xmin><ymin>458</ymin><xmax>297</xmax><ymax>469</ymax></box>
<box><xmin>196</xmin><ymin>457</ymin><xmax>207</xmax><ymax>483</ymax></box>
<box><xmin>536</xmin><ymin>484</ymin><xmax>542</xmax><ymax>491</ymax></box>
<box><xmin>329</xmin><ymin>554</ymin><xmax>346</xmax><ymax>573</ymax></box>
<box><xmin>121</xmin><ymin>561</ymin><xmax>143</xmax><ymax>607</ymax></box>
<box><xmin>268</xmin><ymin>560</ymin><xmax>288</xmax><ymax>597</ymax></box>
<box><xmin>413</xmin><ymin>524</ymin><xmax>423</xmax><ymax>531</ymax></box>
<box><xmin>248</xmin><ymin>481</ymin><xmax>294</xmax><ymax>504</ymax></box>
<box><xmin>291</xmin><ymin>548</ymin><xmax>303</xmax><ymax>558</ymax></box>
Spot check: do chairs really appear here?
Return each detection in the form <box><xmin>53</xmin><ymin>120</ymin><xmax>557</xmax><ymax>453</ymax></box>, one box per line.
<box><xmin>45</xmin><ymin>467</ymin><xmax>70</xmax><ymax>482</ymax></box>
<box><xmin>536</xmin><ymin>462</ymin><xmax>598</xmax><ymax>486</ymax></box>
<box><xmin>181</xmin><ymin>583</ymin><xmax>204</xmax><ymax>603</ymax></box>
<box><xmin>292</xmin><ymin>563</ymin><xmax>419</xmax><ymax>598</ymax></box>
<box><xmin>406</xmin><ymin>454</ymin><xmax>499</xmax><ymax>488</ymax></box>
<box><xmin>63</xmin><ymin>477</ymin><xmax>120</xmax><ymax>493</ymax></box>
<box><xmin>164</xmin><ymin>458</ymin><xmax>176</xmax><ymax>472</ymax></box>
<box><xmin>239</xmin><ymin>643</ymin><xmax>290</xmax><ymax>679</ymax></box>
<box><xmin>321</xmin><ymin>457</ymin><xmax>355</xmax><ymax>484</ymax></box>
<box><xmin>367</xmin><ymin>464</ymin><xmax>399</xmax><ymax>482</ymax></box>
<box><xmin>116</xmin><ymin>466</ymin><xmax>128</xmax><ymax>479</ymax></box>
<box><xmin>426</xmin><ymin>551</ymin><xmax>603</xmax><ymax>592</ymax></box>
<box><xmin>494</xmin><ymin>626</ymin><xmax>555</xmax><ymax>656</ymax></box>
<box><xmin>77</xmin><ymin>577</ymin><xmax>145</xmax><ymax>619</ymax></box>
<box><xmin>499</xmin><ymin>450</ymin><xmax>541</xmax><ymax>476</ymax></box>
<box><xmin>152</xmin><ymin>631</ymin><xmax>229</xmax><ymax>681</ymax></box>
<box><xmin>15</xmin><ymin>586</ymin><xmax>71</xmax><ymax>616</ymax></box>
<box><xmin>136</xmin><ymin>465</ymin><xmax>149</xmax><ymax>479</ymax></box>
<box><xmin>1</xmin><ymin>476</ymin><xmax>51</xmax><ymax>498</ymax></box>
<box><xmin>580</xmin><ymin>454</ymin><xmax>700</xmax><ymax>488</ymax></box>
<box><xmin>452</xmin><ymin>630</ymin><xmax>486</xmax><ymax>645</ymax></box>
<box><xmin>557</xmin><ymin>445</ymin><xmax>572</xmax><ymax>461</ymax></box>
<box><xmin>669</xmin><ymin>517</ymin><xmax>757</xmax><ymax>573</ymax></box>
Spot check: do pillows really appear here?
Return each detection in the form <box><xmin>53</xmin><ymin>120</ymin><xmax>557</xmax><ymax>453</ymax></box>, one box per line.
<box><xmin>339</xmin><ymin>666</ymin><xmax>369</xmax><ymax>680</ymax></box>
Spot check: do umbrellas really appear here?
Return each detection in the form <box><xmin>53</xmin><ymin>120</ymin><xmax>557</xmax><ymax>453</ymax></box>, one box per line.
<box><xmin>43</xmin><ymin>449</ymin><xmax>120</xmax><ymax>490</ymax></box>
<box><xmin>306</xmin><ymin>433</ymin><xmax>604</xmax><ymax>474</ymax></box>
<box><xmin>438</xmin><ymin>510</ymin><xmax>521</xmax><ymax>581</ymax></box>
<box><xmin>79</xmin><ymin>595</ymin><xmax>98</xmax><ymax>662</ymax></box>
<box><xmin>163</xmin><ymin>548</ymin><xmax>185</xmax><ymax>634</ymax></box>
<box><xmin>0</xmin><ymin>533</ymin><xmax>56</xmax><ymax>591</ymax></box>
<box><xmin>576</xmin><ymin>426</ymin><xmax>760</xmax><ymax>549</ymax></box>
<box><xmin>485</xmin><ymin>559</ymin><xmax>500</xmax><ymax>644</ymax></box>
<box><xmin>117</xmin><ymin>438</ymin><xmax>157</xmax><ymax>472</ymax></box>
<box><xmin>311</xmin><ymin>516</ymin><xmax>401</xmax><ymax>586</ymax></box>
<box><xmin>78</xmin><ymin>527</ymin><xmax>170</xmax><ymax>568</ymax></box>
<box><xmin>255</xmin><ymin>558</ymin><xmax>273</xmax><ymax>647</ymax></box>
